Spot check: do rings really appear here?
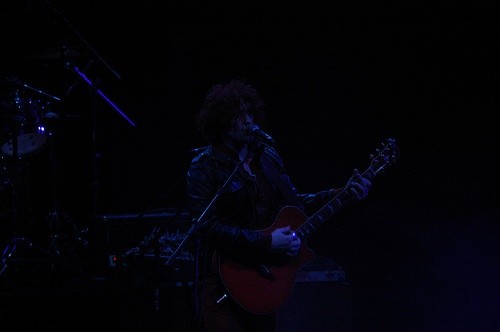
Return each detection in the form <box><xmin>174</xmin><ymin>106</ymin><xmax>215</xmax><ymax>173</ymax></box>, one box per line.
<box><xmin>287</xmin><ymin>234</ymin><xmax>292</xmax><ymax>241</ymax></box>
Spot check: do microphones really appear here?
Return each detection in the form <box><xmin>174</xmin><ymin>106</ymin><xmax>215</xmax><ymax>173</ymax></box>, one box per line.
<box><xmin>249</xmin><ymin>125</ymin><xmax>275</xmax><ymax>146</ymax></box>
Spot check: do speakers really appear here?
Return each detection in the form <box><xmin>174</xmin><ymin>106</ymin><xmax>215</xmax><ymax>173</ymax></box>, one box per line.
<box><xmin>273</xmin><ymin>273</ymin><xmax>353</xmax><ymax>332</ymax></box>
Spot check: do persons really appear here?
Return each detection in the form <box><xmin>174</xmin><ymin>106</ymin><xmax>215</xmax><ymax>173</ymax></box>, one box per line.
<box><xmin>186</xmin><ymin>82</ymin><xmax>373</xmax><ymax>332</ymax></box>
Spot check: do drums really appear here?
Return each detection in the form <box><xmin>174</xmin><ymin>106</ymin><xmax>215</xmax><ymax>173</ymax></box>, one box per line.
<box><xmin>0</xmin><ymin>99</ymin><xmax>50</xmax><ymax>158</ymax></box>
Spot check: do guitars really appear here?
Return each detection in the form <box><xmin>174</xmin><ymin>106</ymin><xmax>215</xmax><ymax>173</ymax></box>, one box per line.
<box><xmin>209</xmin><ymin>136</ymin><xmax>405</xmax><ymax>319</ymax></box>
